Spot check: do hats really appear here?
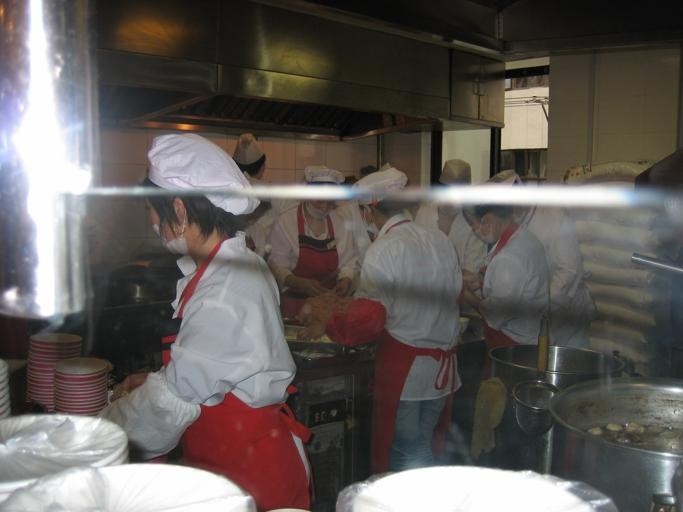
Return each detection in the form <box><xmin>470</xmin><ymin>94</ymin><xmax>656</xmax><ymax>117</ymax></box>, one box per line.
<box><xmin>147</xmin><ymin>133</ymin><xmax>260</xmax><ymax>216</ymax></box>
<box><xmin>485</xmin><ymin>169</ymin><xmax>521</xmax><ymax>185</ymax></box>
<box><xmin>439</xmin><ymin>159</ymin><xmax>472</xmax><ymax>185</ymax></box>
<box><xmin>233</xmin><ymin>133</ymin><xmax>265</xmax><ymax>165</ymax></box>
<box><xmin>304</xmin><ymin>166</ymin><xmax>345</xmax><ymax>184</ymax></box>
<box><xmin>351</xmin><ymin>168</ymin><xmax>409</xmax><ymax>206</ymax></box>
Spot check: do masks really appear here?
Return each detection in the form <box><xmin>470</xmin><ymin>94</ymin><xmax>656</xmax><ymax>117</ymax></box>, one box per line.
<box><xmin>474</xmin><ymin>221</ymin><xmax>498</xmax><ymax>244</ymax></box>
<box><xmin>361</xmin><ymin>215</ymin><xmax>380</xmax><ymax>235</ymax></box>
<box><xmin>306</xmin><ymin>202</ymin><xmax>333</xmax><ymax>221</ymax></box>
<box><xmin>153</xmin><ymin>208</ymin><xmax>189</xmax><ymax>256</ymax></box>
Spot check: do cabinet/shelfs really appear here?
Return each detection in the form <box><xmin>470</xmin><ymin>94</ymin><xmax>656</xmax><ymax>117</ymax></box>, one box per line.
<box><xmin>451</xmin><ymin>47</ymin><xmax>506</xmax><ymax>129</ymax></box>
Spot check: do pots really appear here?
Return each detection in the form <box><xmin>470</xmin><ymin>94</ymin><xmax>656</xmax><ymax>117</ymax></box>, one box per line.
<box><xmin>545</xmin><ymin>376</ymin><xmax>682</xmax><ymax>512</ymax></box>
<box><xmin>106</xmin><ymin>265</ymin><xmax>159</xmax><ymax>307</ymax></box>
<box><xmin>487</xmin><ymin>344</ymin><xmax>625</xmax><ymax>472</ymax></box>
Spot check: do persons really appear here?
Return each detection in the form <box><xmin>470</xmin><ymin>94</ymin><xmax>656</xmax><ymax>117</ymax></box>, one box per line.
<box><xmin>416</xmin><ymin>158</ymin><xmax>595</xmax><ymax>371</ymax></box>
<box><xmin>270</xmin><ymin>164</ymin><xmax>377</xmax><ymax>318</ymax></box>
<box><xmin>232</xmin><ymin>134</ymin><xmax>281</xmax><ymax>256</ymax></box>
<box><xmin>98</xmin><ymin>134</ymin><xmax>313</xmax><ymax>511</ymax></box>
<box><xmin>297</xmin><ymin>166</ymin><xmax>463</xmax><ymax>476</ymax></box>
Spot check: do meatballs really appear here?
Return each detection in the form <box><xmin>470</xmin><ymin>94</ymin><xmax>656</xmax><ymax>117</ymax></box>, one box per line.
<box><xmin>606</xmin><ymin>424</ymin><xmax>623</xmax><ymax>432</ymax></box>
<box><xmin>587</xmin><ymin>427</ymin><xmax>602</xmax><ymax>436</ymax></box>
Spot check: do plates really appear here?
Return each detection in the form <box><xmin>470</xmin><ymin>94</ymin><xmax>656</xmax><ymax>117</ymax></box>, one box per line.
<box><xmin>23</xmin><ymin>331</ymin><xmax>110</xmax><ymax>418</ymax></box>
<box><xmin>0</xmin><ymin>357</ymin><xmax>12</xmax><ymax>422</ymax></box>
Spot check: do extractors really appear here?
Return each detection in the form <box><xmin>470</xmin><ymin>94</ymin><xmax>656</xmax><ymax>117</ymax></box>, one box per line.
<box><xmin>99</xmin><ymin>84</ymin><xmax>380</xmax><ymax>138</ymax></box>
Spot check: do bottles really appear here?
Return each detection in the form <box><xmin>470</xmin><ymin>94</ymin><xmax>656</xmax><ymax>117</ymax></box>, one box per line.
<box><xmin>648</xmin><ymin>491</ymin><xmax>678</xmax><ymax>511</ymax></box>
